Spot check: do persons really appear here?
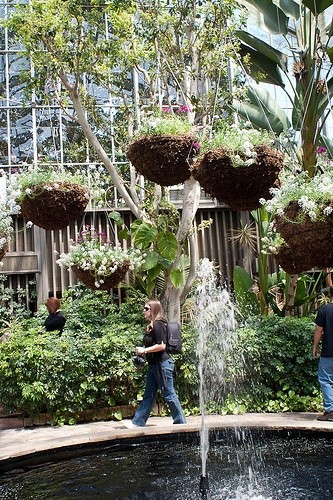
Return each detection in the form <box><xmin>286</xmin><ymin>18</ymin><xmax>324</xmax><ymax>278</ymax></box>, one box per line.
<box><xmin>313</xmin><ymin>287</ymin><xmax>333</xmax><ymax>421</ymax></box>
<box><xmin>119</xmin><ymin>300</ymin><xmax>186</xmax><ymax>424</ymax></box>
<box><xmin>42</xmin><ymin>297</ymin><xmax>67</xmax><ymax>338</ymax></box>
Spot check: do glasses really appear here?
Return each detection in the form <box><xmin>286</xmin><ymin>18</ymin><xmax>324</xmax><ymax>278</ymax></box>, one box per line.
<box><xmin>144</xmin><ymin>307</ymin><xmax>151</xmax><ymax>311</ymax></box>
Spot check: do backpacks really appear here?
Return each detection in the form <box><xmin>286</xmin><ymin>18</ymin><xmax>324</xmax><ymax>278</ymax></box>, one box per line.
<box><xmin>151</xmin><ymin>318</ymin><xmax>183</xmax><ymax>355</ymax></box>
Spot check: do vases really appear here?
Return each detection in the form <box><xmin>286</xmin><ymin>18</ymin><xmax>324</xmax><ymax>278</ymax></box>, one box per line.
<box><xmin>21</xmin><ymin>181</ymin><xmax>90</xmax><ymax>231</ymax></box>
<box><xmin>268</xmin><ymin>199</ymin><xmax>333</xmax><ymax>275</ymax></box>
<box><xmin>70</xmin><ymin>260</ymin><xmax>129</xmax><ymax>291</ymax></box>
<box><xmin>125</xmin><ymin>134</ymin><xmax>200</xmax><ymax>186</ymax></box>
<box><xmin>191</xmin><ymin>146</ymin><xmax>284</xmax><ymax>211</ymax></box>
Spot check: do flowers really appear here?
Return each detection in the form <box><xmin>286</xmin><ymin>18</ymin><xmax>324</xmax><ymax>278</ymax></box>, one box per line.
<box><xmin>0</xmin><ymin>112</ymin><xmax>333</xmax><ymax>288</ymax></box>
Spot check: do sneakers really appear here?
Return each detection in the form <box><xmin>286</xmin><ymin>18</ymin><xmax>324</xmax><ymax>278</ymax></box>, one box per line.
<box><xmin>317</xmin><ymin>412</ymin><xmax>333</xmax><ymax>421</ymax></box>
<box><xmin>120</xmin><ymin>420</ymin><xmax>138</xmax><ymax>429</ymax></box>
